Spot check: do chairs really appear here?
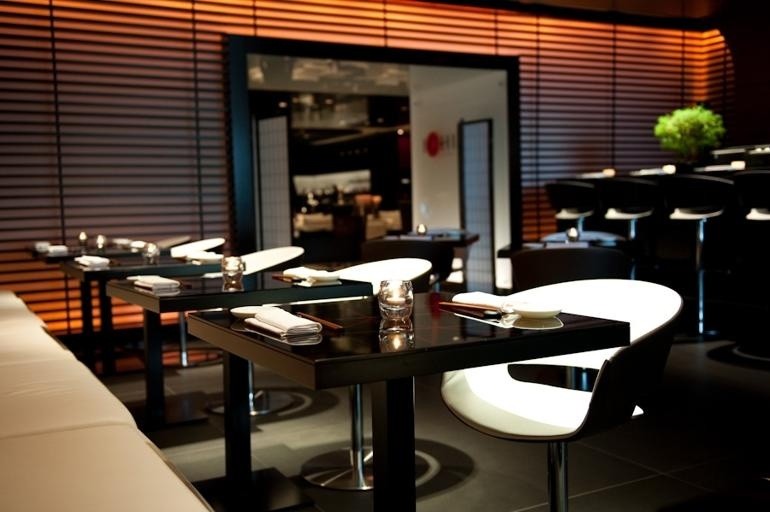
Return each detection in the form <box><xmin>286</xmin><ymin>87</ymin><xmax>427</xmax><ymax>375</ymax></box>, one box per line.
<box><xmin>435</xmin><ymin>275</ymin><xmax>688</xmax><ymax>511</ymax></box>
<box><xmin>283</xmin><ymin>144</ymin><xmax>767</xmax><ymax>374</ymax></box>
<box><xmin>56</xmin><ymin>251</ymin><xmax>229</xmax><ymax>430</ymax></box>
<box><xmin>196</xmin><ymin>244</ymin><xmax>309</xmax><ymax>421</ymax></box>
<box><xmin>296</xmin><ymin>253</ymin><xmax>437</xmax><ymax>492</ymax></box>
<box><xmin>168</xmin><ymin>234</ymin><xmax>227</xmax><ymax>373</ymax></box>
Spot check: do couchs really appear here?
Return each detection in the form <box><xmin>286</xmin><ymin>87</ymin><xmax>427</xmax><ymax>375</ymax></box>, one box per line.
<box><xmin>0</xmin><ymin>287</ymin><xmax>214</xmax><ymax>512</ymax></box>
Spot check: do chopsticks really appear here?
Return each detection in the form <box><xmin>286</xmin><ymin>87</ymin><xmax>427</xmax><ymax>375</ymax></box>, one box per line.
<box><xmin>439</xmin><ymin>300</ymin><xmax>501</xmax><ymax>312</ymax></box>
<box><xmin>297</xmin><ymin>310</ymin><xmax>345</xmax><ymax>330</ymax></box>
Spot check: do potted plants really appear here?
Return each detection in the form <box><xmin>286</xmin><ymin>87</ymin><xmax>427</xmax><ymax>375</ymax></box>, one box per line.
<box><xmin>653</xmin><ymin>104</ymin><xmax>728</xmax><ymax>172</ymax></box>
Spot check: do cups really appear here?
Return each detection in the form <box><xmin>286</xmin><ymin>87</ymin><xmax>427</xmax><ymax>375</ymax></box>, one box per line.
<box><xmin>142</xmin><ymin>242</ymin><xmax>161</xmax><ymax>264</ymax></box>
<box><xmin>222</xmin><ymin>282</ymin><xmax>243</xmax><ymax>292</ymax></box>
<box><xmin>379</xmin><ymin>319</ymin><xmax>417</xmax><ymax>353</ymax></box>
<box><xmin>221</xmin><ymin>255</ymin><xmax>244</xmax><ymax>282</ymax></box>
<box><xmin>378</xmin><ymin>280</ymin><xmax>414</xmax><ymax>318</ymax></box>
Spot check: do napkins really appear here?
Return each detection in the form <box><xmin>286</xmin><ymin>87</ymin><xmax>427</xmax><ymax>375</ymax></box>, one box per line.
<box><xmin>127</xmin><ymin>273</ymin><xmax>182</xmax><ymax>296</ymax></box>
<box><xmin>73</xmin><ymin>254</ymin><xmax>110</xmax><ymax>271</ymax></box>
<box><xmin>44</xmin><ymin>244</ymin><xmax>69</xmax><ymax>258</ymax></box>
<box><xmin>240</xmin><ymin>300</ymin><xmax>322</xmax><ymax>341</ymax></box>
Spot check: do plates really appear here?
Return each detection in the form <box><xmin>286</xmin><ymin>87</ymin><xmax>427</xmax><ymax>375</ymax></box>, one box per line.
<box><xmin>513</xmin><ymin>305</ymin><xmax>561</xmax><ymax>318</ymax></box>
<box><xmin>229</xmin><ymin>318</ymin><xmax>250</xmax><ymax>335</ymax></box>
<box><xmin>125</xmin><ymin>275</ymin><xmax>159</xmax><ymax>281</ymax></box>
<box><xmin>502</xmin><ymin>313</ymin><xmax>564</xmax><ymax>331</ymax></box>
<box><xmin>230</xmin><ymin>306</ymin><xmax>263</xmax><ymax>318</ymax></box>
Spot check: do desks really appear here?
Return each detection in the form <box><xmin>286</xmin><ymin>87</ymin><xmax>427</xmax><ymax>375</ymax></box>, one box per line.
<box><xmin>26</xmin><ymin>241</ymin><xmax>150</xmax><ymax>379</ymax></box>
<box><xmin>178</xmin><ymin>286</ymin><xmax>634</xmax><ymax>512</ymax></box>
<box><xmin>101</xmin><ymin>261</ymin><xmax>376</xmax><ymax>510</ymax></box>
